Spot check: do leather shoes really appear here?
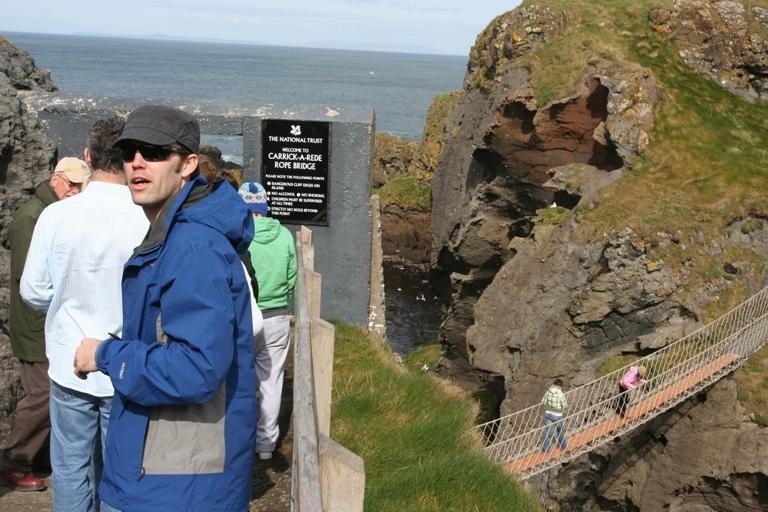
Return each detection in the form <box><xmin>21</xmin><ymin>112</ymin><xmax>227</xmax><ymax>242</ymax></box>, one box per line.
<box><xmin>0</xmin><ymin>471</ymin><xmax>47</xmax><ymax>491</ymax></box>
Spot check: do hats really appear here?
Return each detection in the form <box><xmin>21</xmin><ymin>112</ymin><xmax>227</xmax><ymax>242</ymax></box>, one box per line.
<box><xmin>238</xmin><ymin>182</ymin><xmax>268</xmax><ymax>214</ymax></box>
<box><xmin>639</xmin><ymin>366</ymin><xmax>646</xmax><ymax>376</ymax></box>
<box><xmin>112</xmin><ymin>105</ymin><xmax>200</xmax><ymax>153</ymax></box>
<box><xmin>55</xmin><ymin>156</ymin><xmax>91</xmax><ymax>183</ymax></box>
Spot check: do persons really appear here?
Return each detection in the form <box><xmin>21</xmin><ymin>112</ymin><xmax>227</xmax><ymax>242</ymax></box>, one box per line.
<box><xmin>541</xmin><ymin>379</ymin><xmax>568</xmax><ymax>454</ymax></box>
<box><xmin>616</xmin><ymin>365</ymin><xmax>648</xmax><ymax>419</ymax></box>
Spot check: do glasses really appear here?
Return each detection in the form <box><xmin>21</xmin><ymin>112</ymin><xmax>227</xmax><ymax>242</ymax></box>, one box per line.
<box><xmin>118</xmin><ymin>141</ymin><xmax>186</xmax><ymax>161</ymax></box>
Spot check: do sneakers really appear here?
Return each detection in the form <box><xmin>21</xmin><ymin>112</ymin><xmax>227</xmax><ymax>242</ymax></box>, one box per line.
<box><xmin>254</xmin><ymin>448</ymin><xmax>272</xmax><ymax>460</ymax></box>
